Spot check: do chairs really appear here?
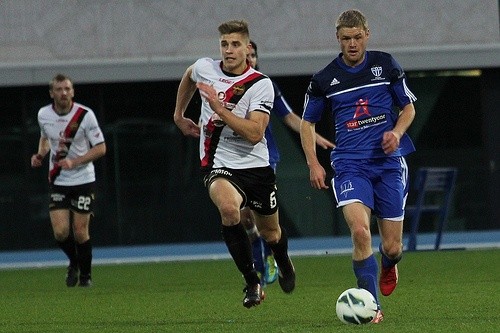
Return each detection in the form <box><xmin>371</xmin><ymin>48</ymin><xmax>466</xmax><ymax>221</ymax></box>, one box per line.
<box><xmin>405</xmin><ymin>167</ymin><xmax>457</xmax><ymax>252</ymax></box>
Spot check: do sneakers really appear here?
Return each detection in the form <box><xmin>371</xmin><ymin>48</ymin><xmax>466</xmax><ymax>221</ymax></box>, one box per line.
<box><xmin>273</xmin><ymin>251</ymin><xmax>296</xmax><ymax>292</ymax></box>
<box><xmin>255</xmin><ymin>270</ymin><xmax>267</xmax><ymax>288</ymax></box>
<box><xmin>379</xmin><ymin>261</ymin><xmax>398</xmax><ymax>296</ymax></box>
<box><xmin>243</xmin><ymin>283</ymin><xmax>265</xmax><ymax>309</ymax></box>
<box><xmin>264</xmin><ymin>254</ymin><xmax>278</xmax><ymax>284</ymax></box>
<box><xmin>371</xmin><ymin>310</ymin><xmax>384</xmax><ymax>324</ymax></box>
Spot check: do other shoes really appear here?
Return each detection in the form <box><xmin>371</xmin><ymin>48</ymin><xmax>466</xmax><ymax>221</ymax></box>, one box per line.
<box><xmin>79</xmin><ymin>273</ymin><xmax>92</xmax><ymax>287</ymax></box>
<box><xmin>66</xmin><ymin>266</ymin><xmax>79</xmax><ymax>287</ymax></box>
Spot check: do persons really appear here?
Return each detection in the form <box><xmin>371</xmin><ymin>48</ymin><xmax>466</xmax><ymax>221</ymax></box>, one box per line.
<box><xmin>300</xmin><ymin>10</ymin><xmax>417</xmax><ymax>323</ymax></box>
<box><xmin>31</xmin><ymin>73</ymin><xmax>106</xmax><ymax>288</ymax></box>
<box><xmin>173</xmin><ymin>19</ymin><xmax>335</xmax><ymax>308</ymax></box>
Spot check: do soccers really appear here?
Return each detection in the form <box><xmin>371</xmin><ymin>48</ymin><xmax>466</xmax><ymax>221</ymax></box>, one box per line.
<box><xmin>335</xmin><ymin>288</ymin><xmax>378</xmax><ymax>326</ymax></box>
